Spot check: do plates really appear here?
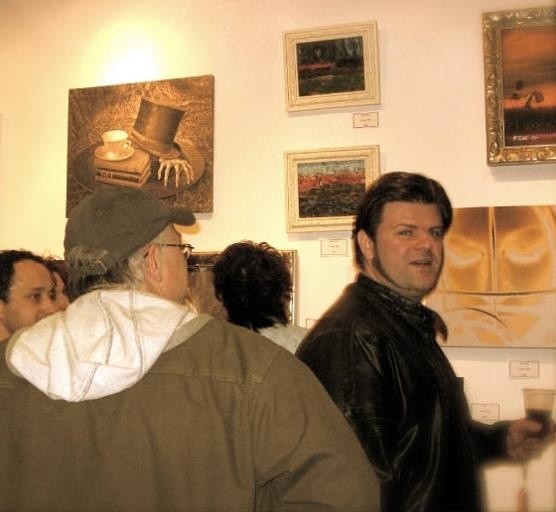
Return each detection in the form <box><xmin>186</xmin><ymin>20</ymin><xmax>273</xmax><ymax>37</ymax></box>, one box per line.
<box><xmin>94</xmin><ymin>145</ymin><xmax>136</xmax><ymax>163</ymax></box>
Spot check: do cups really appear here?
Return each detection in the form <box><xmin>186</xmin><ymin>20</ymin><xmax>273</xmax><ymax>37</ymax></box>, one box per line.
<box><xmin>102</xmin><ymin>130</ymin><xmax>132</xmax><ymax>157</ymax></box>
<box><xmin>523</xmin><ymin>387</ymin><xmax>555</xmax><ymax>437</ymax></box>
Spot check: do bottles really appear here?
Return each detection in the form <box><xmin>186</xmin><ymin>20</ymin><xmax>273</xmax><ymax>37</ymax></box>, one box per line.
<box><xmin>516</xmin><ymin>462</ymin><xmax>532</xmax><ymax>511</ymax></box>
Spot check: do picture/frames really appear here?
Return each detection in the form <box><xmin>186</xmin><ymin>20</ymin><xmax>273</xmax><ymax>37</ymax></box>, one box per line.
<box><xmin>480</xmin><ymin>4</ymin><xmax>556</xmax><ymax>165</ymax></box>
<box><xmin>181</xmin><ymin>248</ymin><xmax>299</xmax><ymax>325</ymax></box>
<box><xmin>281</xmin><ymin>18</ymin><xmax>382</xmax><ymax>113</ymax></box>
<box><xmin>282</xmin><ymin>142</ymin><xmax>382</xmax><ymax>235</ymax></box>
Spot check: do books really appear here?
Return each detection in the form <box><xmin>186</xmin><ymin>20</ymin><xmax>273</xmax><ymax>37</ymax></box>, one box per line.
<box><xmin>94</xmin><ymin>148</ymin><xmax>151</xmax><ymax>187</ymax></box>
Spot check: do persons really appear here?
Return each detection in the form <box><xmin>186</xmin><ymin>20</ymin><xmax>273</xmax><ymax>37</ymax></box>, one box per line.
<box><xmin>0</xmin><ymin>187</ymin><xmax>382</xmax><ymax>511</ymax></box>
<box><xmin>211</xmin><ymin>239</ymin><xmax>312</xmax><ymax>354</ymax></box>
<box><xmin>45</xmin><ymin>255</ymin><xmax>70</xmax><ymax>311</ymax></box>
<box><xmin>297</xmin><ymin>170</ymin><xmax>555</xmax><ymax>512</ymax></box>
<box><xmin>0</xmin><ymin>249</ymin><xmax>56</xmax><ymax>342</ymax></box>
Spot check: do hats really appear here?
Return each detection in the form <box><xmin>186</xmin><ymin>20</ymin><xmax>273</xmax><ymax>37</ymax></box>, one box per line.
<box><xmin>63</xmin><ymin>185</ymin><xmax>198</xmax><ymax>278</ymax></box>
